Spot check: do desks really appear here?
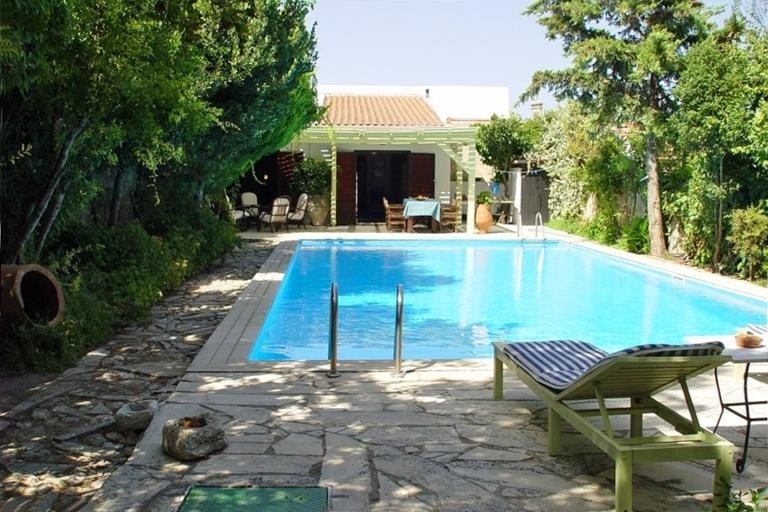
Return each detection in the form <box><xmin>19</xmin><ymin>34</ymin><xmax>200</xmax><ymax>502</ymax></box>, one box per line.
<box><xmin>685</xmin><ymin>334</ymin><xmax>768</xmax><ymax>475</ymax></box>
<box><xmin>403</xmin><ymin>197</ymin><xmax>440</xmax><ymax>233</ymax></box>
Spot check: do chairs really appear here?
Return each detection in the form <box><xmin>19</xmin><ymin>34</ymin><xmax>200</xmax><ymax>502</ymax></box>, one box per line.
<box><xmin>439</xmin><ymin>196</ymin><xmax>461</xmax><ymax>232</ymax></box>
<box><xmin>488</xmin><ymin>336</ymin><xmax>735</xmax><ymax>512</ymax></box>
<box><xmin>383</xmin><ymin>196</ymin><xmax>406</xmax><ymax>232</ymax></box>
<box><xmin>223</xmin><ymin>188</ymin><xmax>308</xmax><ymax>234</ymax></box>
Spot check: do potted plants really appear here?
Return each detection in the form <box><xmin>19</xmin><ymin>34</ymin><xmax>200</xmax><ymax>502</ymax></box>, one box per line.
<box><xmin>475</xmin><ymin>191</ymin><xmax>495</xmax><ymax>233</ymax></box>
<box><xmin>293</xmin><ymin>156</ymin><xmax>342</xmax><ymax>226</ymax></box>
